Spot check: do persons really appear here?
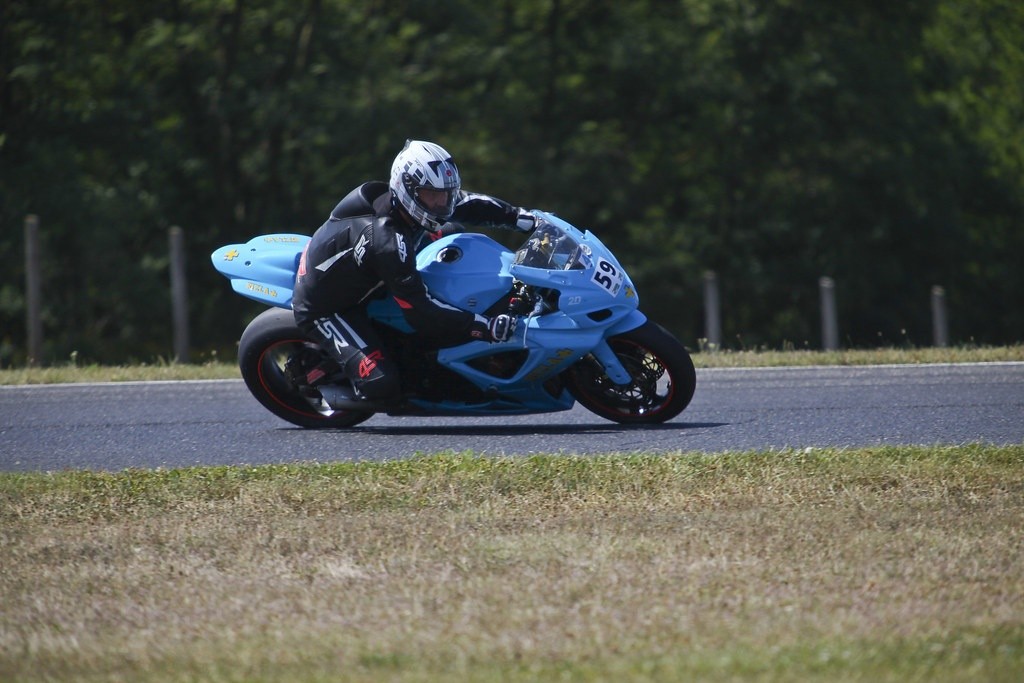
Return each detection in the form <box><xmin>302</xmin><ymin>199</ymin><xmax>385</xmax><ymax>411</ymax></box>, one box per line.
<box><xmin>293</xmin><ymin>139</ymin><xmax>559</xmax><ymax>415</ymax></box>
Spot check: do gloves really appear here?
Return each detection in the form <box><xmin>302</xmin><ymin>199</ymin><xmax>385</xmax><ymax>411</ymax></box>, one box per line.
<box><xmin>514</xmin><ymin>206</ymin><xmax>560</xmax><ymax>237</ymax></box>
<box><xmin>467</xmin><ymin>313</ymin><xmax>517</xmax><ymax>343</ymax></box>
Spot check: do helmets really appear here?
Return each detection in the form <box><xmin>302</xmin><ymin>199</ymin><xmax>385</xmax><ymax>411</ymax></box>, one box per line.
<box><xmin>388</xmin><ymin>137</ymin><xmax>461</xmax><ymax>233</ymax></box>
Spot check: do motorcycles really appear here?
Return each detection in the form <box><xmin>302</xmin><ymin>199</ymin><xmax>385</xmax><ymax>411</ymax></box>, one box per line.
<box><xmin>210</xmin><ymin>208</ymin><xmax>698</xmax><ymax>426</ymax></box>
